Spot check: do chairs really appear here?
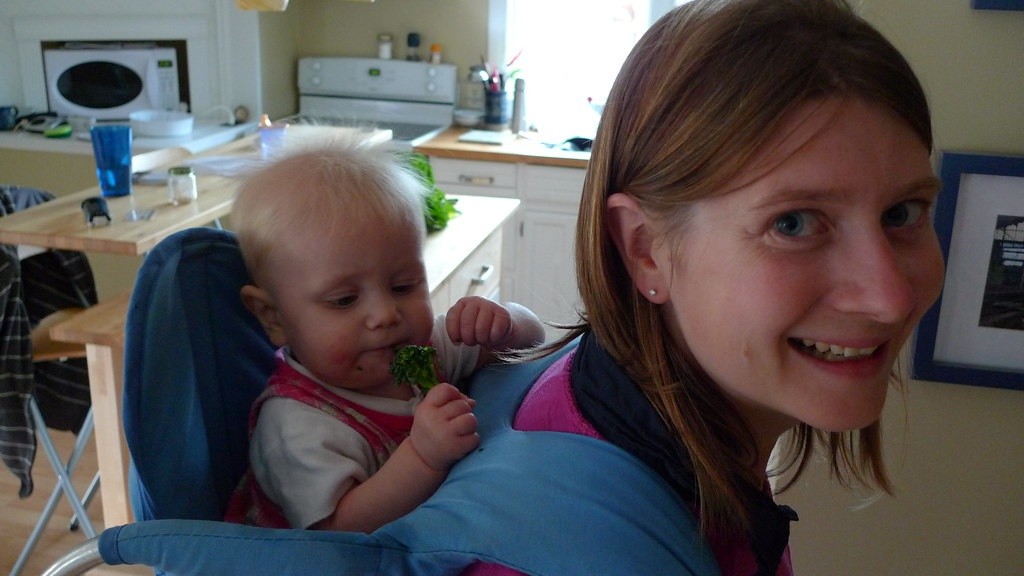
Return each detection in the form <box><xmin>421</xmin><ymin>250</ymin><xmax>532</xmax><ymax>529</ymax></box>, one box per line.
<box><xmin>0</xmin><ymin>183</ymin><xmax>100</xmax><ymax>576</ymax></box>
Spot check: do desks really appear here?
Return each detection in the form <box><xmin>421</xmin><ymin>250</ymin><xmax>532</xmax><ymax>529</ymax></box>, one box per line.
<box><xmin>0</xmin><ymin>130</ymin><xmax>265</xmax><ymax>264</ymax></box>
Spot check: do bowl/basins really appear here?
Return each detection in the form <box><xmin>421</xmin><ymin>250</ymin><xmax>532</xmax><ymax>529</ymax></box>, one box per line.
<box><xmin>128</xmin><ymin>111</ymin><xmax>194</xmax><ymax>137</ymax></box>
<box><xmin>454</xmin><ymin>109</ymin><xmax>485</xmax><ymax>127</ymax></box>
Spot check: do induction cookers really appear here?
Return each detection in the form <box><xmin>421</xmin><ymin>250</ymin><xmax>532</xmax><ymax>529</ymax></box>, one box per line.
<box><xmin>270</xmin><ymin>57</ymin><xmax>457</xmax><ymax>154</ymax></box>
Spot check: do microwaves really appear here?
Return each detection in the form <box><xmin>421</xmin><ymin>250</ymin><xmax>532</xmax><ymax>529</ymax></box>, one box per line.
<box><xmin>41</xmin><ymin>42</ymin><xmax>189</xmax><ymax>122</ymax></box>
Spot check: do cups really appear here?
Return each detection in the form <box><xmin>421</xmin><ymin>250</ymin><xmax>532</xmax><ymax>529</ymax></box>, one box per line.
<box><xmin>0</xmin><ymin>105</ymin><xmax>19</xmax><ymax>131</ymax></box>
<box><xmin>484</xmin><ymin>91</ymin><xmax>507</xmax><ymax>124</ymax></box>
<box><xmin>89</xmin><ymin>125</ymin><xmax>133</xmax><ymax>197</ymax></box>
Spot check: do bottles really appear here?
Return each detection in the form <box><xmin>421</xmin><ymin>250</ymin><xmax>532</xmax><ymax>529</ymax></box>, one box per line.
<box><xmin>407</xmin><ymin>33</ymin><xmax>422</xmax><ymax>62</ymax></box>
<box><xmin>429</xmin><ymin>44</ymin><xmax>443</xmax><ymax>63</ymax></box>
<box><xmin>377</xmin><ymin>33</ymin><xmax>393</xmax><ymax>60</ymax></box>
<box><xmin>168</xmin><ymin>167</ymin><xmax>197</xmax><ymax>205</ymax></box>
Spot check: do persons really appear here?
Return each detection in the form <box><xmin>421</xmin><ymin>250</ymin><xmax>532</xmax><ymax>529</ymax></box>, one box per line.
<box><xmin>459</xmin><ymin>0</ymin><xmax>945</xmax><ymax>576</ymax></box>
<box><xmin>223</xmin><ymin>113</ymin><xmax>547</xmax><ymax>535</ymax></box>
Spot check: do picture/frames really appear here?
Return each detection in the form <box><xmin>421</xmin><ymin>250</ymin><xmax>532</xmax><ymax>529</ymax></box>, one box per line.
<box><xmin>907</xmin><ymin>148</ymin><xmax>1024</xmax><ymax>394</ymax></box>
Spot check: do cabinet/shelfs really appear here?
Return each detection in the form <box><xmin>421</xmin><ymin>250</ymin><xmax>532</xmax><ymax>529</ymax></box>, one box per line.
<box><xmin>448</xmin><ymin>227</ymin><xmax>500</xmax><ymax>305</ymax></box>
<box><xmin>515</xmin><ymin>161</ymin><xmax>588</xmax><ymax>343</ymax></box>
<box><xmin>429</xmin><ymin>156</ymin><xmax>520</xmax><ymax>304</ymax></box>
